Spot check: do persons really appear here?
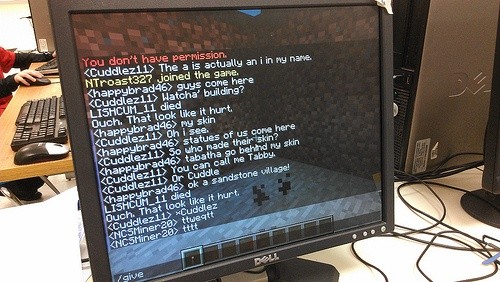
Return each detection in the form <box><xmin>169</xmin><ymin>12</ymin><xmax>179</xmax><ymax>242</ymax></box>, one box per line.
<box><xmin>0</xmin><ymin>44</ymin><xmax>56</xmax><ymax>203</ymax></box>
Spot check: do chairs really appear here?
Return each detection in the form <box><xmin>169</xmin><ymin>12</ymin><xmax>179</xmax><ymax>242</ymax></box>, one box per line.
<box><xmin>0</xmin><ymin>173</ymin><xmax>60</xmax><ymax>205</ymax></box>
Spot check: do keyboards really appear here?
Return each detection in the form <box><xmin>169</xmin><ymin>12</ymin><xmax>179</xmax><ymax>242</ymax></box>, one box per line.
<box><xmin>36</xmin><ymin>57</ymin><xmax>59</xmax><ymax>75</ymax></box>
<box><xmin>10</xmin><ymin>94</ymin><xmax>68</xmax><ymax>152</ymax></box>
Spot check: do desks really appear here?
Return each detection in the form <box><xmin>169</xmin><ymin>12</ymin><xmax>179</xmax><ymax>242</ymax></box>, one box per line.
<box><xmin>0</xmin><ymin>162</ymin><xmax>500</xmax><ymax>282</ymax></box>
<box><xmin>0</xmin><ymin>54</ymin><xmax>77</xmax><ymax>206</ymax></box>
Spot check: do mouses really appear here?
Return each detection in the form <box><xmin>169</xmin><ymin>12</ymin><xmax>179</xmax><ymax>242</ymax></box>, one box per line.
<box><xmin>20</xmin><ymin>76</ymin><xmax>51</xmax><ymax>87</ymax></box>
<box><xmin>14</xmin><ymin>141</ymin><xmax>69</xmax><ymax>165</ymax></box>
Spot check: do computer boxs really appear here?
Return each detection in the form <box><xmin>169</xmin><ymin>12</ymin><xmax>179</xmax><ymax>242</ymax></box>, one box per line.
<box><xmin>396</xmin><ymin>0</ymin><xmax>500</xmax><ymax>228</ymax></box>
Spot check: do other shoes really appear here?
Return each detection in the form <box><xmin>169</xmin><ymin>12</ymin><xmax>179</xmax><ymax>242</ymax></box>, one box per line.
<box><xmin>20</xmin><ymin>192</ymin><xmax>43</xmax><ymax>202</ymax></box>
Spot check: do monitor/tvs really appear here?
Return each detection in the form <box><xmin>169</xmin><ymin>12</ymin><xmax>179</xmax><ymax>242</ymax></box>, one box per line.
<box><xmin>49</xmin><ymin>0</ymin><xmax>395</xmax><ymax>282</ymax></box>
